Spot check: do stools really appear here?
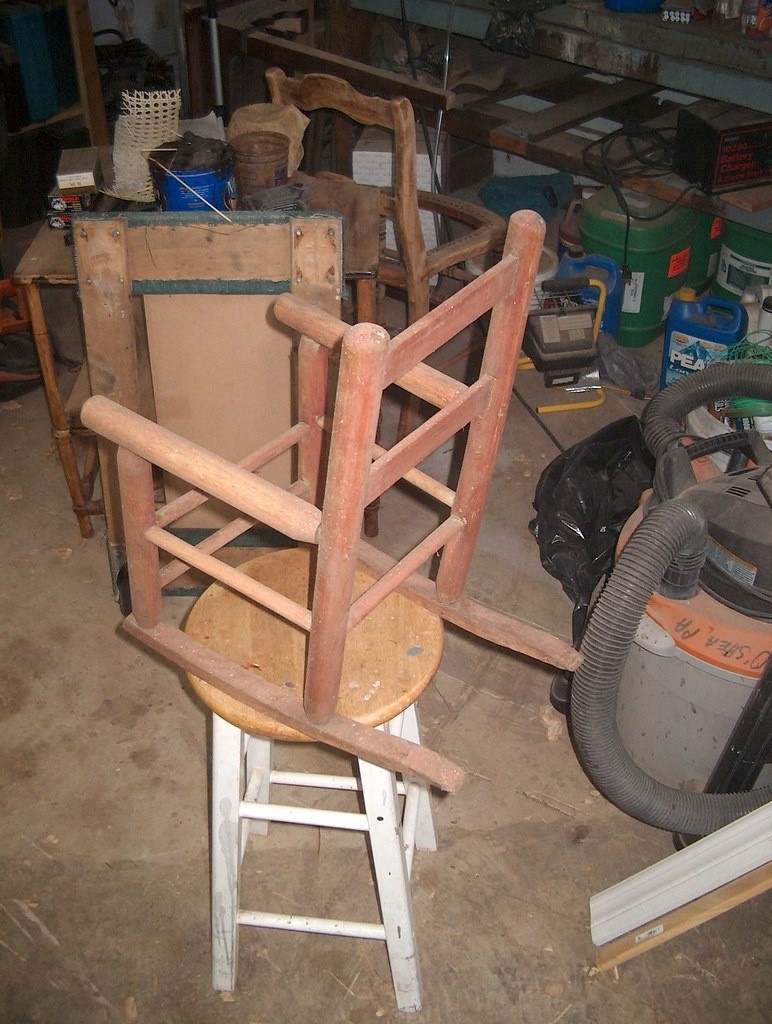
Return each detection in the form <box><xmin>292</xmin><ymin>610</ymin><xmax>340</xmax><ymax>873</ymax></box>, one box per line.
<box><xmin>185</xmin><ymin>547</ymin><xmax>445</xmax><ymax>1013</ymax></box>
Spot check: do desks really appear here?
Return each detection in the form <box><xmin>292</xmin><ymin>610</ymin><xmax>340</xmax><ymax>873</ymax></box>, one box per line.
<box><xmin>12</xmin><ymin>177</ymin><xmax>381</xmax><ymax>539</ymax></box>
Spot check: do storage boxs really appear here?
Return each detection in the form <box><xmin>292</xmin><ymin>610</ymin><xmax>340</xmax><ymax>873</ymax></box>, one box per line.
<box><xmin>46</xmin><ymin>148</ymin><xmax>104</xmax><ymax>230</ymax></box>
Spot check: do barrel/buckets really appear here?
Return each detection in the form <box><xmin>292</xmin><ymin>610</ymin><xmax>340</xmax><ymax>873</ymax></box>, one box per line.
<box><xmin>660</xmin><ymin>287</ymin><xmax>748</xmax><ymax>391</ymax></box>
<box><xmin>739</xmin><ymin>283</ymin><xmax>772</xmax><ymax>349</ymax></box>
<box><xmin>557</xmin><ymin>188</ymin><xmax>597</xmax><ymax>260</ymax></box>
<box><xmin>558</xmin><ymin>244</ymin><xmax>621</xmax><ymax>346</ymax></box>
<box><xmin>709</xmin><ymin>219</ymin><xmax>772</xmax><ymax>316</ymax></box>
<box><xmin>578</xmin><ymin>185</ymin><xmax>699</xmax><ymax>347</ymax></box>
<box><xmin>687</xmin><ymin>208</ymin><xmax>721</xmax><ymax>296</ymax></box>
<box><xmin>230</xmin><ymin>131</ymin><xmax>291</xmax><ymax>198</ymax></box>
<box><xmin>465</xmin><ymin>241</ymin><xmax>559</xmax><ymax>311</ymax></box>
